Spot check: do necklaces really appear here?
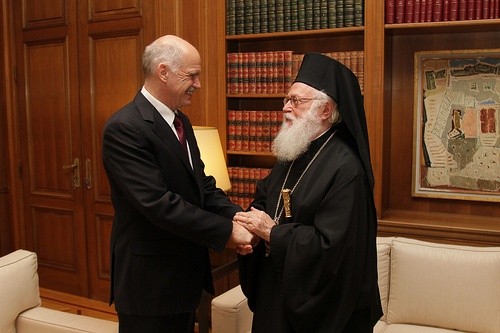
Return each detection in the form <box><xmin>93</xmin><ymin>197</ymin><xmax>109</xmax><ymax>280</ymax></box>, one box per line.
<box><xmin>264</xmin><ymin>127</ymin><xmax>340</xmax><ymax>256</ymax></box>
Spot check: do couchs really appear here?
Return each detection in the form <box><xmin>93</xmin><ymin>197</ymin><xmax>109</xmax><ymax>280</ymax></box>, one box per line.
<box><xmin>0</xmin><ymin>249</ymin><xmax>119</xmax><ymax>333</ymax></box>
<box><xmin>211</xmin><ymin>236</ymin><xmax>500</xmax><ymax>333</ymax></box>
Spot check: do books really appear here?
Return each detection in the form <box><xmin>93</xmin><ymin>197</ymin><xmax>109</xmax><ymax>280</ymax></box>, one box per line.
<box><xmin>384</xmin><ymin>1</ymin><xmax>500</xmax><ymax>25</ymax></box>
<box><xmin>222</xmin><ymin>0</ymin><xmax>366</xmax><ymax>238</ymax></box>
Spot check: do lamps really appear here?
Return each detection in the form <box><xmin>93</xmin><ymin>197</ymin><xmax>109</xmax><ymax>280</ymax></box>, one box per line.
<box><xmin>193</xmin><ymin>125</ymin><xmax>232</xmax><ymax>191</ymax></box>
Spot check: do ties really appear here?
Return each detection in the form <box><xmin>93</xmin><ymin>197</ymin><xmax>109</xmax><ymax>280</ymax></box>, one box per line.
<box><xmin>173</xmin><ymin>113</ymin><xmax>189</xmax><ymax>161</ymax></box>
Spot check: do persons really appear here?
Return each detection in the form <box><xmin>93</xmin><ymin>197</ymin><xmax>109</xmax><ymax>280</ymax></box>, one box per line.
<box><xmin>100</xmin><ymin>35</ymin><xmax>257</xmax><ymax>332</ymax></box>
<box><xmin>230</xmin><ymin>53</ymin><xmax>384</xmax><ymax>333</ymax></box>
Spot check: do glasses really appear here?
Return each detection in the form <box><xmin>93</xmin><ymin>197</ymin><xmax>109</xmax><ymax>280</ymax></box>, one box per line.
<box><xmin>283</xmin><ymin>96</ymin><xmax>326</xmax><ymax>108</ymax></box>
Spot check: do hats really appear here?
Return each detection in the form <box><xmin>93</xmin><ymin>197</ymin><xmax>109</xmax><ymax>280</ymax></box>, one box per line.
<box><xmin>294</xmin><ymin>51</ymin><xmax>375</xmax><ymax>195</ymax></box>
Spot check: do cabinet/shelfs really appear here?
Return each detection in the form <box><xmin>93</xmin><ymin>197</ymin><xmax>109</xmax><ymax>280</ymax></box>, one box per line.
<box><xmin>223</xmin><ymin>0</ymin><xmax>500</xmax><ymax>242</ymax></box>
